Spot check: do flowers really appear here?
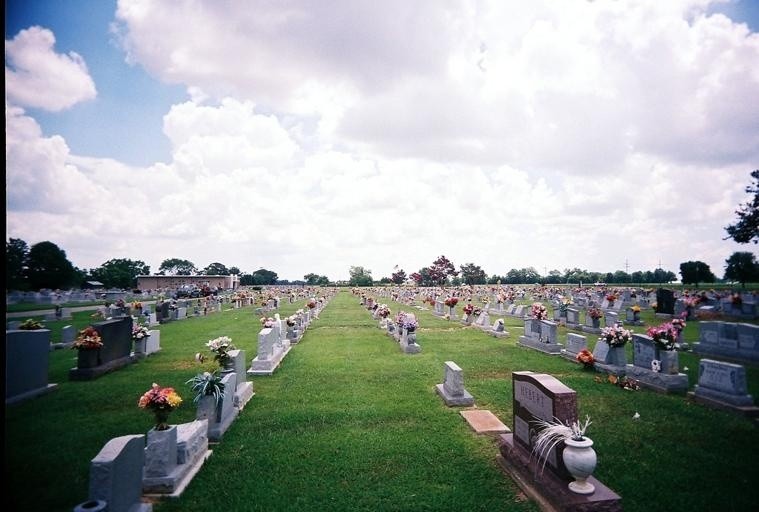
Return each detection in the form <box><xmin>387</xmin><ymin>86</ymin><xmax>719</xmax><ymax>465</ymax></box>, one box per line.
<box><xmin>576</xmin><ymin>349</ymin><xmax>594</xmax><ymax>364</ymax></box>
<box><xmin>377</xmin><ymin>295</ymin><xmax>701</xmax><ymax>332</ymax></box>
<box><xmin>597</xmin><ymin>322</ymin><xmax>634</xmax><ymax>349</ymax></box>
<box><xmin>70</xmin><ymin>298</ymin><xmax>274</xmax><ymax>430</ymax></box>
<box><xmin>645</xmin><ymin>321</ymin><xmax>680</xmax><ymax>353</ymax></box>
